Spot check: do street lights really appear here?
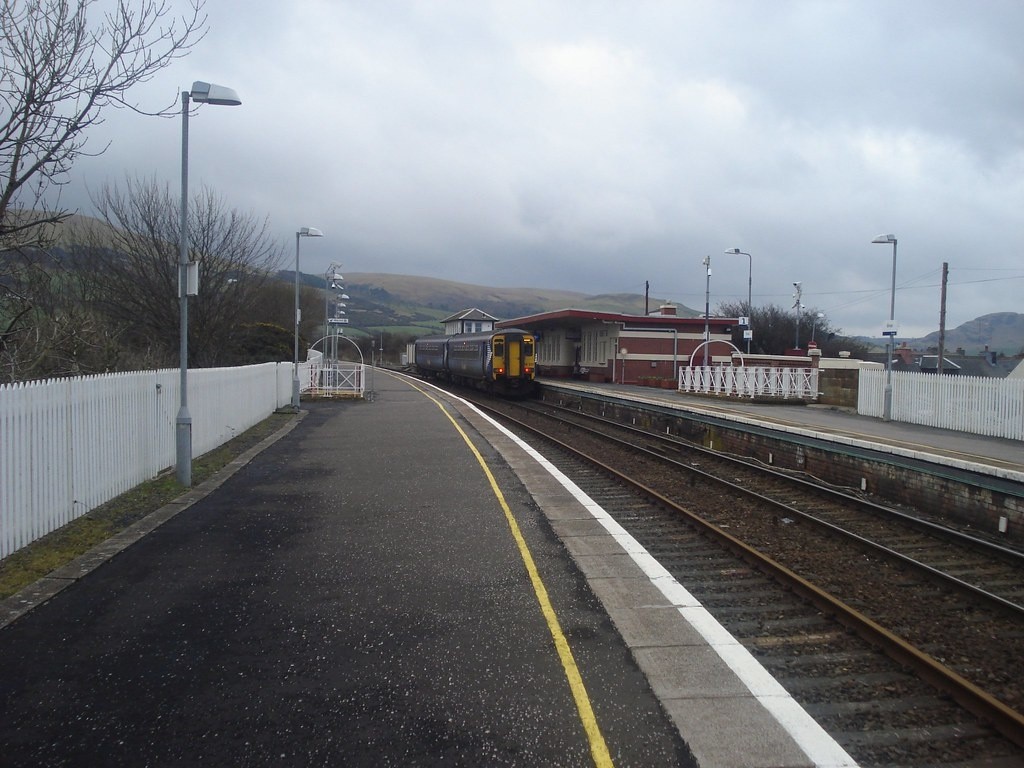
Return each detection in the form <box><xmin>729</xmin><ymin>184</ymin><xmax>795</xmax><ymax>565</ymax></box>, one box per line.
<box><xmin>723</xmin><ymin>247</ymin><xmax>752</xmax><ymax>355</ymax></box>
<box><xmin>290</xmin><ymin>226</ymin><xmax>325</xmax><ymax>410</ymax></box>
<box><xmin>871</xmin><ymin>234</ymin><xmax>899</xmax><ymax>423</ymax></box>
<box><xmin>330</xmin><ymin>292</ymin><xmax>351</xmax><ymax>387</ymax></box>
<box><xmin>324</xmin><ymin>273</ymin><xmax>344</xmax><ymax>387</ymax></box>
<box><xmin>702</xmin><ymin>255</ymin><xmax>711</xmax><ymax>366</ymax></box>
<box><xmin>812</xmin><ymin>312</ymin><xmax>825</xmax><ymax>342</ymax></box>
<box><xmin>174</xmin><ymin>80</ymin><xmax>243</xmax><ymax>489</ymax></box>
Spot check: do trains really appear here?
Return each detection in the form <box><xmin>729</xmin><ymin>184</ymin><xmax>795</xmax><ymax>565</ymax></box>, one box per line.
<box><xmin>414</xmin><ymin>328</ymin><xmax>537</xmax><ymax>397</ymax></box>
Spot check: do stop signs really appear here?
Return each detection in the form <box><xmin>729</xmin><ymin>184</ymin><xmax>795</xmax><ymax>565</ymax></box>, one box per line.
<box><xmin>808</xmin><ymin>341</ymin><xmax>817</xmax><ymax>350</ymax></box>
